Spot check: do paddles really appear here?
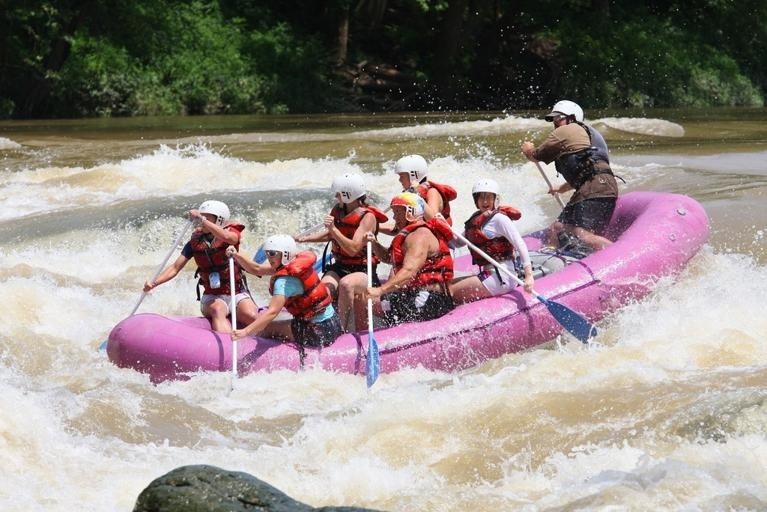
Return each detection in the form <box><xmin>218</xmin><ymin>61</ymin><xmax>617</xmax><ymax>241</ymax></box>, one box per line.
<box><xmin>450</xmin><ymin>228</ymin><xmax>597</xmax><ymax>345</ymax></box>
<box><xmin>100</xmin><ymin>213</ymin><xmax>197</xmax><ymax>352</ymax></box>
<box><xmin>365</xmin><ymin>241</ymin><xmax>379</xmax><ymax>386</ymax></box>
<box><xmin>253</xmin><ymin>221</ymin><xmax>327</xmax><ymax>264</ymax></box>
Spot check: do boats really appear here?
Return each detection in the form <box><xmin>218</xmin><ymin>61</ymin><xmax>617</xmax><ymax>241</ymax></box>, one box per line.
<box><xmin>101</xmin><ymin>188</ymin><xmax>710</xmax><ymax>388</ymax></box>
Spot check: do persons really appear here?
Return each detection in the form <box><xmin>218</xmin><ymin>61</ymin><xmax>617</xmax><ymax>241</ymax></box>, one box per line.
<box><xmin>520</xmin><ymin>100</ymin><xmax>618</xmax><ymax>250</ymax></box>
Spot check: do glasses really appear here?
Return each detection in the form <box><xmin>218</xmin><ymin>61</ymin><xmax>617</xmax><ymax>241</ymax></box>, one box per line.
<box><xmin>266</xmin><ymin>250</ymin><xmax>279</xmax><ymax>257</ymax></box>
<box><xmin>552</xmin><ymin>115</ymin><xmax>568</xmax><ymax>123</ymax></box>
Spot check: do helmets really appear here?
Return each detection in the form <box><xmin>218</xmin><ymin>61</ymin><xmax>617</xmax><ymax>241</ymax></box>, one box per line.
<box><xmin>199</xmin><ymin>200</ymin><xmax>230</xmax><ymax>229</ymax></box>
<box><xmin>394</xmin><ymin>155</ymin><xmax>428</xmax><ymax>186</ymax></box>
<box><xmin>471</xmin><ymin>178</ymin><xmax>502</xmax><ymax>209</ymax></box>
<box><xmin>330</xmin><ymin>172</ymin><xmax>365</xmax><ymax>205</ymax></box>
<box><xmin>545</xmin><ymin>99</ymin><xmax>584</xmax><ymax>125</ymax></box>
<box><xmin>390</xmin><ymin>192</ymin><xmax>424</xmax><ymax>223</ymax></box>
<box><xmin>262</xmin><ymin>234</ymin><xmax>296</xmax><ymax>266</ymax></box>
